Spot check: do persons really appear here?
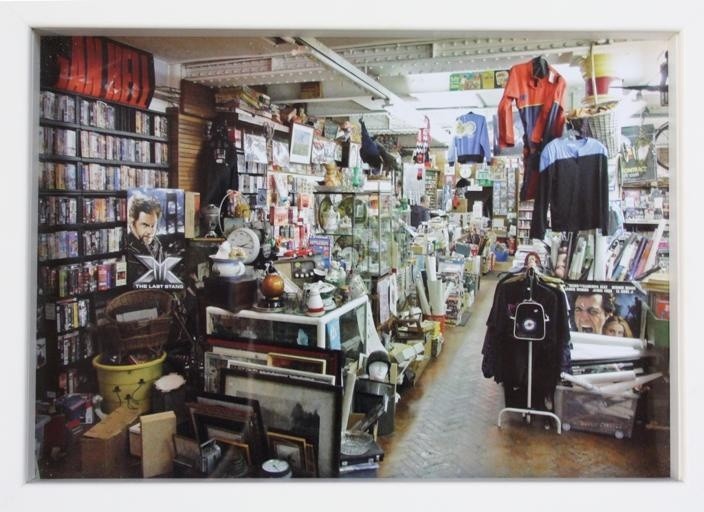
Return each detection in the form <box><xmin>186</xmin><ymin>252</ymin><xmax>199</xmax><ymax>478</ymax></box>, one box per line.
<box><xmin>411</xmin><ymin>195</ymin><xmax>431</xmax><ymax>228</ymax></box>
<box><xmin>602</xmin><ymin>316</ymin><xmax>633</xmax><ymax>337</ymax></box>
<box><xmin>127</xmin><ymin>194</ymin><xmax>163</xmax><ymax>264</ymax></box>
<box><xmin>572</xmin><ymin>292</ymin><xmax>617</xmax><ymax>335</ymax></box>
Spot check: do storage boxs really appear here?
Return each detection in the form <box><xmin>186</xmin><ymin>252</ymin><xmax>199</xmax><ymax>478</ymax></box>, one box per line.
<box><xmin>553</xmin><ymin>382</ymin><xmax>641</xmax><ymax>440</ymax></box>
<box><xmin>34</xmin><ymin>404</ymin><xmax>144</xmax><ymax>477</ymax></box>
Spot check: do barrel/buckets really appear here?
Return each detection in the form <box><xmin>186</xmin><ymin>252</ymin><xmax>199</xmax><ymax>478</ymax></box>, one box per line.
<box><xmin>90</xmin><ymin>351</ymin><xmax>169</xmax><ymax>417</ymax></box>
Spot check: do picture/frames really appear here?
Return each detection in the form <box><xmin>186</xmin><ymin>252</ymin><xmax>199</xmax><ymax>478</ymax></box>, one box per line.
<box><xmin>189</xmin><ymin>391</ymin><xmax>317</xmax><ymax>477</ymax></box>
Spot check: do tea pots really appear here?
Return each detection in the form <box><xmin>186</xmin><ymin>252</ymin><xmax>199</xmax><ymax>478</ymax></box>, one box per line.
<box><xmin>323</xmin><ymin>205</ymin><xmax>341</xmax><ymax>234</ymax></box>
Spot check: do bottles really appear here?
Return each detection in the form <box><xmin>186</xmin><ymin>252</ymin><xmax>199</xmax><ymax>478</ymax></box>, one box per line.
<box><xmin>305</xmin><ymin>288</ymin><xmax>326</xmax><ymax>317</ymax></box>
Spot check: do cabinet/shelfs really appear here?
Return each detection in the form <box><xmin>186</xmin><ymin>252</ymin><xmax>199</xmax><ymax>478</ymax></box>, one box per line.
<box><xmin>35</xmin><ymin>86</ymin><xmax>669</xmax><ymax>415</ymax></box>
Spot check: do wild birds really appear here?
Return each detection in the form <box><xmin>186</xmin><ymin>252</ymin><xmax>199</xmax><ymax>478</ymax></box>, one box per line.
<box><xmin>358</xmin><ymin>116</ymin><xmax>401</xmax><ymax>178</ymax></box>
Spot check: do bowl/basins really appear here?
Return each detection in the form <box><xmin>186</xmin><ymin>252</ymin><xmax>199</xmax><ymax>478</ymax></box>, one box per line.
<box><xmin>340</xmin><ymin>431</ymin><xmax>373</xmax><ymax>456</ymax></box>
